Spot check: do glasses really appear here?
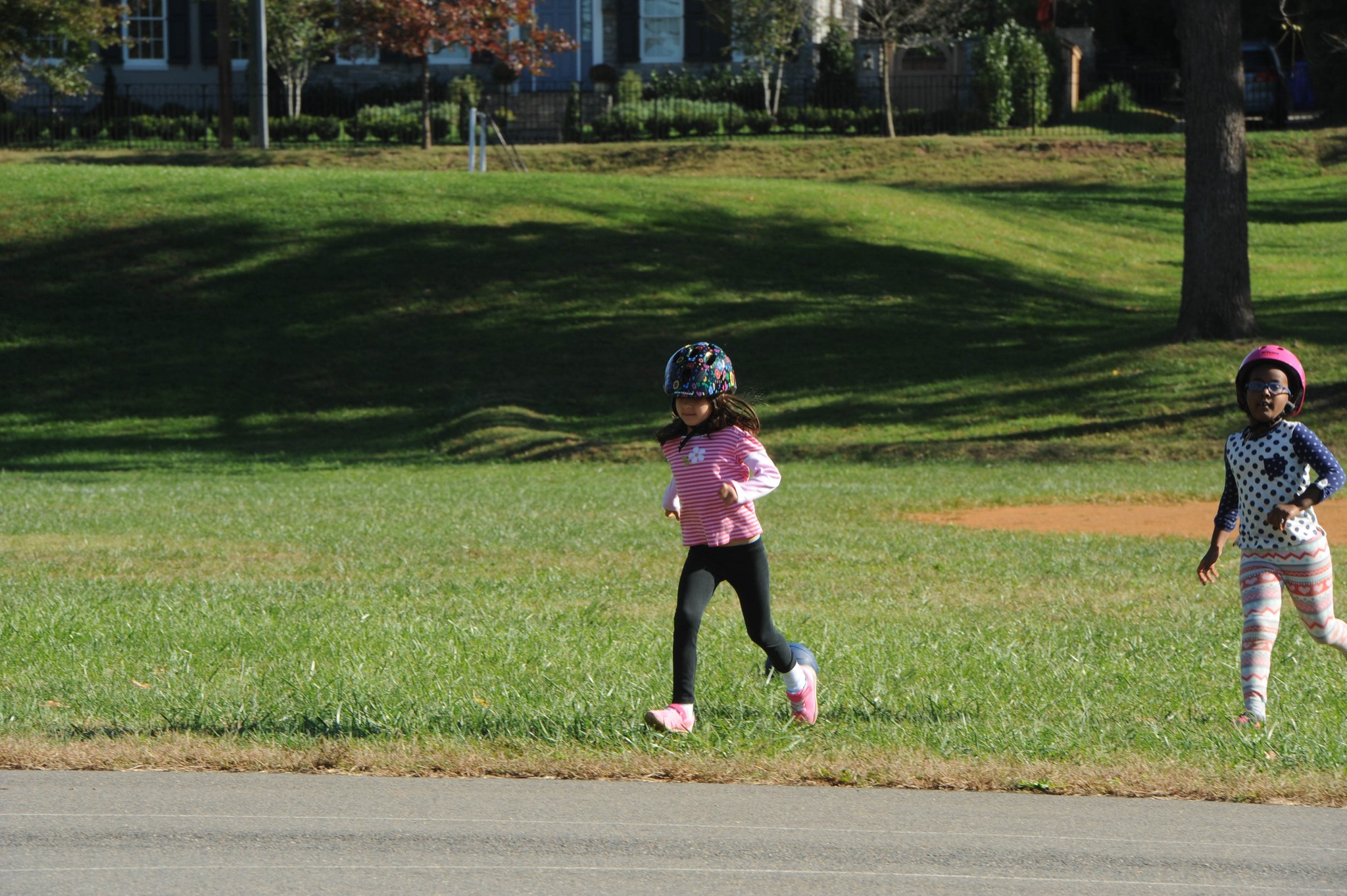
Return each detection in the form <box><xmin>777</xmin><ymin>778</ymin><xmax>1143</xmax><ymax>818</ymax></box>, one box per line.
<box><xmin>1244</xmin><ymin>381</ymin><xmax>1291</xmax><ymax>396</ymax></box>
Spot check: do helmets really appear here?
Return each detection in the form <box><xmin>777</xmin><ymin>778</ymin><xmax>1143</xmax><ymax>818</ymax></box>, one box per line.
<box><xmin>1236</xmin><ymin>345</ymin><xmax>1305</xmax><ymax>417</ymax></box>
<box><xmin>664</xmin><ymin>342</ymin><xmax>737</xmax><ymax>397</ymax></box>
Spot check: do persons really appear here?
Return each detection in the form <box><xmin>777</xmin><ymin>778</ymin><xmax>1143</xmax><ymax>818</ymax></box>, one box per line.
<box><xmin>643</xmin><ymin>341</ymin><xmax>819</xmax><ymax>737</ymax></box>
<box><xmin>1196</xmin><ymin>346</ymin><xmax>1346</xmax><ymax>729</ymax></box>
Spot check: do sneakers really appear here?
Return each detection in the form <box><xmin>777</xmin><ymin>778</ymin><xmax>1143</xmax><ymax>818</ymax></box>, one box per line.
<box><xmin>786</xmin><ymin>665</ymin><xmax>818</xmax><ymax>726</ymax></box>
<box><xmin>645</xmin><ymin>703</ymin><xmax>695</xmax><ymax>738</ymax></box>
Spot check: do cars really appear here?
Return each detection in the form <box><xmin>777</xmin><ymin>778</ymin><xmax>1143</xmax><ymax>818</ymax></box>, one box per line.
<box><xmin>1241</xmin><ymin>38</ymin><xmax>1296</xmax><ymax>124</ymax></box>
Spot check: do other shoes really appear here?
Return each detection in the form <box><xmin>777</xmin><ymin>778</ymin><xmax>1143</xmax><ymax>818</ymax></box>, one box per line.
<box><xmin>1229</xmin><ymin>711</ymin><xmax>1262</xmax><ymax>729</ymax></box>
